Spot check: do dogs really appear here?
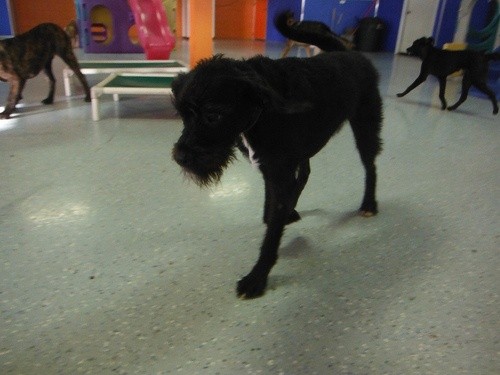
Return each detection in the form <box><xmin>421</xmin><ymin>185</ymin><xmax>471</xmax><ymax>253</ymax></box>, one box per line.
<box><xmin>169</xmin><ymin>6</ymin><xmax>386</xmax><ymax>300</ymax></box>
<box><xmin>396</xmin><ymin>36</ymin><xmax>500</xmax><ymax>114</ymax></box>
<box><xmin>0</xmin><ymin>22</ymin><xmax>91</xmax><ymax>119</ymax></box>
